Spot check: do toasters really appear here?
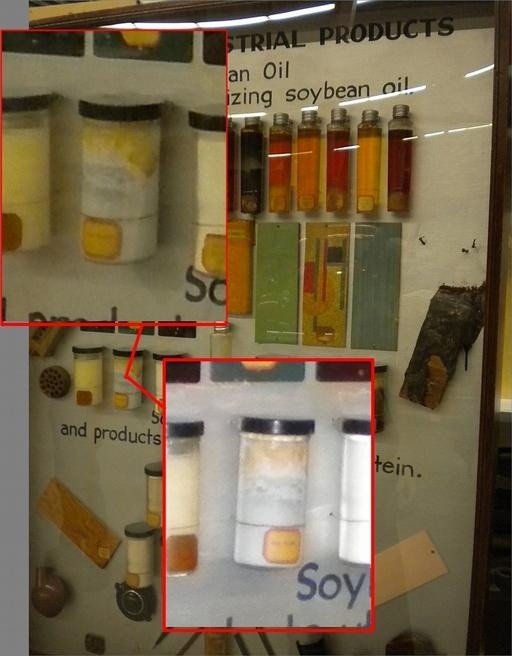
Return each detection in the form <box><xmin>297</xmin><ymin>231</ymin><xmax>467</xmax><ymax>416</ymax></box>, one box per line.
<box><xmin>234</xmin><ymin>416</ymin><xmax>315</xmax><ymax>569</ymax></box>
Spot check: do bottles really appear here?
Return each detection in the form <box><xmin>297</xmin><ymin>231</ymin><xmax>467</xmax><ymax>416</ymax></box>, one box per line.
<box><xmin>338</xmin><ymin>416</ymin><xmax>372</xmax><ymax>565</ymax></box>
<box><xmin>72</xmin><ymin>344</ymin><xmax>105</xmax><ymax>406</ymax></box>
<box><xmin>373</xmin><ymin>364</ymin><xmax>388</xmax><ymax>432</ymax></box>
<box><xmin>229</xmin><ymin>106</ymin><xmax>412</xmax><ymax>215</ymax></box>
<box><xmin>166</xmin><ymin>422</ymin><xmax>202</xmax><ymax>577</ymax></box>
<box><xmin>123</xmin><ymin>523</ymin><xmax>152</xmax><ymax>590</ymax></box>
<box><xmin>144</xmin><ymin>460</ymin><xmax>163</xmax><ymax>528</ymax></box>
<box><xmin>0</xmin><ymin>88</ymin><xmax>61</xmax><ymax>255</ymax></box>
<box><xmin>111</xmin><ymin>346</ymin><xmax>146</xmax><ymax>408</ymax></box>
<box><xmin>210</xmin><ymin>322</ymin><xmax>232</xmax><ymax>357</ymax></box>
<box><xmin>188</xmin><ymin>102</ymin><xmax>225</xmax><ymax>281</ymax></box>
<box><xmin>75</xmin><ymin>92</ymin><xmax>168</xmax><ymax>263</ymax></box>
<box><xmin>153</xmin><ymin>351</ymin><xmax>186</xmax><ymax>413</ymax></box>
